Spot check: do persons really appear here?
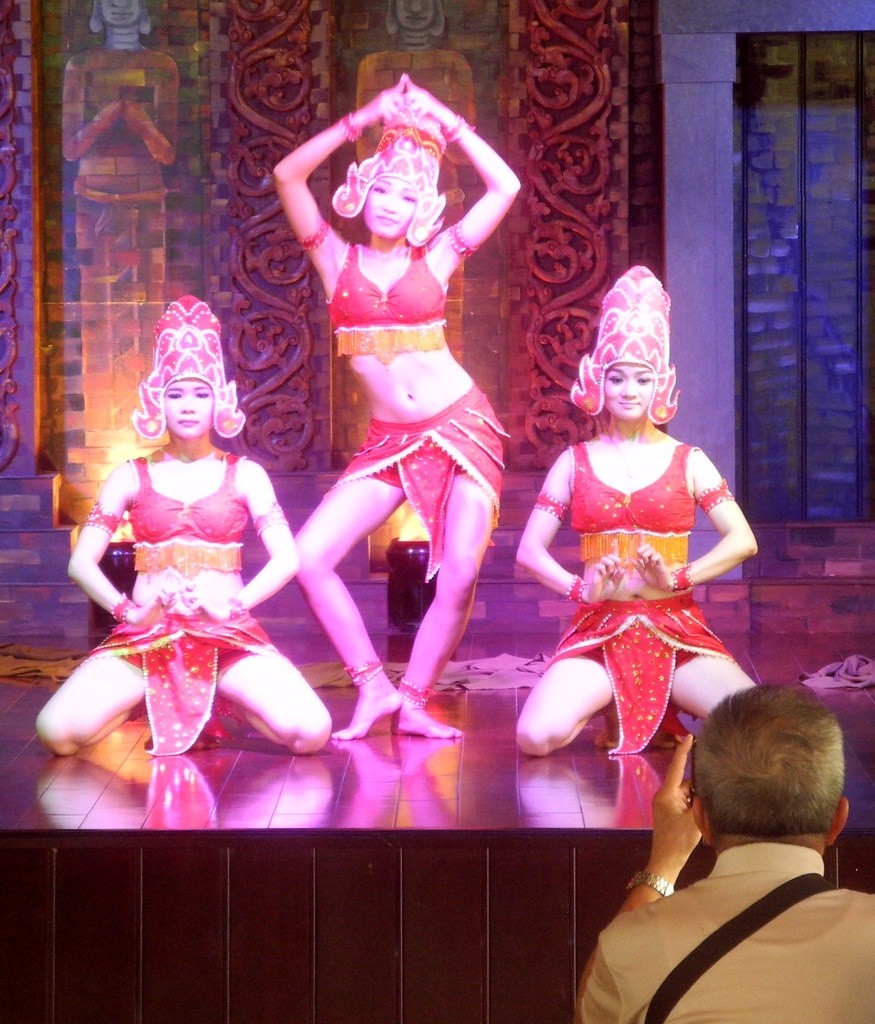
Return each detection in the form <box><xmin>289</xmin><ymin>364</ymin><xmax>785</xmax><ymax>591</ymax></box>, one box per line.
<box><xmin>517</xmin><ymin>757</ymin><xmax>662</xmax><ymax>831</ymax></box>
<box><xmin>331</xmin><ymin>739</ymin><xmax>460</xmax><ymax>829</ymax></box>
<box><xmin>576</xmin><ymin>678</ymin><xmax>875</xmax><ymax>1024</ymax></box>
<box><xmin>270</xmin><ymin>73</ymin><xmax>523</xmax><ymax>741</ymax></box>
<box><xmin>516</xmin><ymin>266</ymin><xmax>759</xmax><ymax>755</ymax></box>
<box><xmin>31</xmin><ymin>295</ymin><xmax>335</xmax><ymax>754</ymax></box>
<box><xmin>37</xmin><ymin>748</ymin><xmax>333</xmax><ymax>829</ymax></box>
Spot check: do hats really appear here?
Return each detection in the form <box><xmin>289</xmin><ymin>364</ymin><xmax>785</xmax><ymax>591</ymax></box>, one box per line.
<box><xmin>333</xmin><ymin>117</ymin><xmax>447</xmax><ymax>248</ymax></box>
<box><xmin>134</xmin><ymin>294</ymin><xmax>246</xmax><ymax>439</ymax></box>
<box><xmin>569</xmin><ymin>265</ymin><xmax>680</xmax><ymax>424</ymax></box>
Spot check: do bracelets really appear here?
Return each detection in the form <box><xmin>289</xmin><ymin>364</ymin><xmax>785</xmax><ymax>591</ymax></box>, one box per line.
<box><xmin>110</xmin><ymin>597</ymin><xmax>134</xmax><ymax>623</ymax></box>
<box><xmin>624</xmin><ymin>871</ymin><xmax>674</xmax><ymax>898</ymax></box>
<box><xmin>339</xmin><ymin>113</ymin><xmax>363</xmax><ymax>144</ymax></box>
<box><xmin>226</xmin><ymin>596</ymin><xmax>248</xmax><ymax>619</ymax></box>
<box><xmin>443</xmin><ymin>114</ymin><xmax>467</xmax><ymax>143</ymax></box>
<box><xmin>670</xmin><ymin>564</ymin><xmax>696</xmax><ymax>594</ymax></box>
<box><xmin>566</xmin><ymin>575</ymin><xmax>587</xmax><ymax>603</ymax></box>
